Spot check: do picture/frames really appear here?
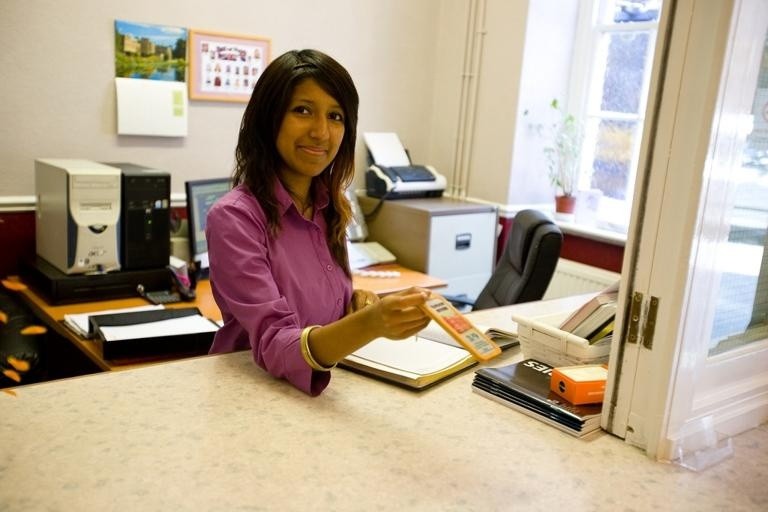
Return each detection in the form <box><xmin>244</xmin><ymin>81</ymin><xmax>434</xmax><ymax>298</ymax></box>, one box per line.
<box><xmin>187</xmin><ymin>29</ymin><xmax>272</xmax><ymax>103</ymax></box>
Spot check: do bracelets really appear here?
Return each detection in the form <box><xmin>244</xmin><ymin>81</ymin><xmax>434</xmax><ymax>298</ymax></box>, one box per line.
<box><xmin>299</xmin><ymin>322</ymin><xmax>338</xmax><ymax>374</ymax></box>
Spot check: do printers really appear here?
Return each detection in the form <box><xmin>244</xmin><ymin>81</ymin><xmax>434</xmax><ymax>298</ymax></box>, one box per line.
<box><xmin>364</xmin><ymin>149</ymin><xmax>447</xmax><ymax>200</ymax></box>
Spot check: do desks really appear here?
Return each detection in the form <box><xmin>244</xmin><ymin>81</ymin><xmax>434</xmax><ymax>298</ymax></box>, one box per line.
<box><xmin>6</xmin><ymin>263</ymin><xmax>450</xmax><ymax>371</ymax></box>
<box><xmin>1</xmin><ymin>292</ymin><xmax>758</xmax><ymax>511</ymax></box>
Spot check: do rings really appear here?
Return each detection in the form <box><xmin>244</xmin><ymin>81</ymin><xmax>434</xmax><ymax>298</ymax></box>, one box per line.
<box><xmin>365</xmin><ymin>300</ymin><xmax>371</xmax><ymax>306</ymax></box>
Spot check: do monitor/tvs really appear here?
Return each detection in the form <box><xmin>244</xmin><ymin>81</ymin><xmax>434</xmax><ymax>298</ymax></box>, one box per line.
<box><xmin>186</xmin><ymin>177</ymin><xmax>237</xmax><ymax>275</ymax></box>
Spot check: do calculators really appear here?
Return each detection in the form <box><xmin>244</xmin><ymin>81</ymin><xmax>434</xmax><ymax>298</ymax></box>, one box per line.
<box><xmin>145</xmin><ymin>291</ymin><xmax>181</xmax><ymax>304</ymax></box>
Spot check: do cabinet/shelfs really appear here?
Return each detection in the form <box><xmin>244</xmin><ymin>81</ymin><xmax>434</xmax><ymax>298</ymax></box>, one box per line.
<box><xmin>357</xmin><ymin>188</ymin><xmax>499</xmax><ymax>303</ymax></box>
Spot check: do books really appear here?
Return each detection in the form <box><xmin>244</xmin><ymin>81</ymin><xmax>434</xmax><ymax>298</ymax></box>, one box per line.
<box><xmin>468</xmin><ymin>357</ymin><xmax>604</xmax><ymax>437</ymax></box>
<box><xmin>559</xmin><ymin>276</ymin><xmax>622</xmax><ymax>342</ymax></box>
<box><xmin>58</xmin><ymin>302</ymin><xmax>225</xmax><ymax>359</ymax></box>
<box><xmin>332</xmin><ymin>325</ymin><xmax>521</xmax><ymax>392</ymax></box>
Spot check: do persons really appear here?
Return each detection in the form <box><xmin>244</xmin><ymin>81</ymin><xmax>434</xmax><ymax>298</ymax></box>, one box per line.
<box><xmin>206</xmin><ymin>49</ymin><xmax>436</xmax><ymax>398</ymax></box>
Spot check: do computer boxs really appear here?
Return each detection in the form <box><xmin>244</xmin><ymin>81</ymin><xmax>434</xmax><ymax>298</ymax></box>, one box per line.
<box><xmin>33</xmin><ymin>158</ymin><xmax>119</xmax><ymax>275</ymax></box>
<box><xmin>105</xmin><ymin>160</ymin><xmax>172</xmax><ymax>271</ymax></box>
